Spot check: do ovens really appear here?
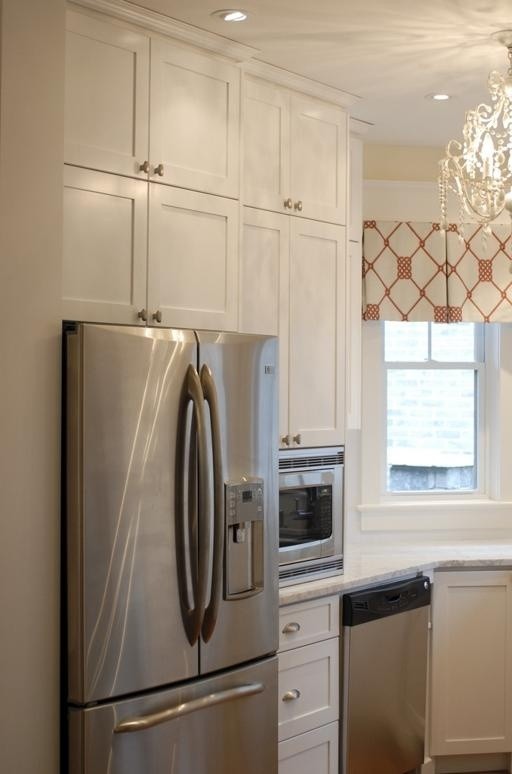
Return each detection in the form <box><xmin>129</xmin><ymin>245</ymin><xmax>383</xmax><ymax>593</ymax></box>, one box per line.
<box><xmin>338</xmin><ymin>579</ymin><xmax>433</xmax><ymax>774</ymax></box>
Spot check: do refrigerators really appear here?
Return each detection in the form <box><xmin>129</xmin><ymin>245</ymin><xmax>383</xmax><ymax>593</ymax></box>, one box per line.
<box><xmin>69</xmin><ymin>322</ymin><xmax>279</xmax><ymax>774</ymax></box>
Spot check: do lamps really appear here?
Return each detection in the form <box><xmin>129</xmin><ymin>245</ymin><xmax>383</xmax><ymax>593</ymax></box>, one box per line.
<box><xmin>440</xmin><ymin>30</ymin><xmax>512</xmax><ymax>258</ymax></box>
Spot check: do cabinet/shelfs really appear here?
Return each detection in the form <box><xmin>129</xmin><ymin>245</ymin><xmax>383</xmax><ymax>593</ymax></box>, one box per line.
<box><xmin>277</xmin><ymin>594</ymin><xmax>340</xmax><ymax>774</ymax></box>
<box><xmin>239</xmin><ymin>206</ymin><xmax>357</xmax><ymax>450</ymax></box>
<box><xmin>64</xmin><ymin>163</ymin><xmax>240</xmax><ymax>340</ymax></box>
<box><xmin>429</xmin><ymin>565</ymin><xmax>512</xmax><ymax>774</ymax></box>
<box><xmin>236</xmin><ymin>63</ymin><xmax>352</xmax><ymax>229</ymax></box>
<box><xmin>66</xmin><ymin>2</ymin><xmax>248</xmax><ymax>203</ymax></box>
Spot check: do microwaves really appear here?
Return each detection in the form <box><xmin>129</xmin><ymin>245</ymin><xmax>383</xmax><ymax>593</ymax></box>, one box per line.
<box><xmin>278</xmin><ymin>448</ymin><xmax>346</xmax><ymax>585</ymax></box>
